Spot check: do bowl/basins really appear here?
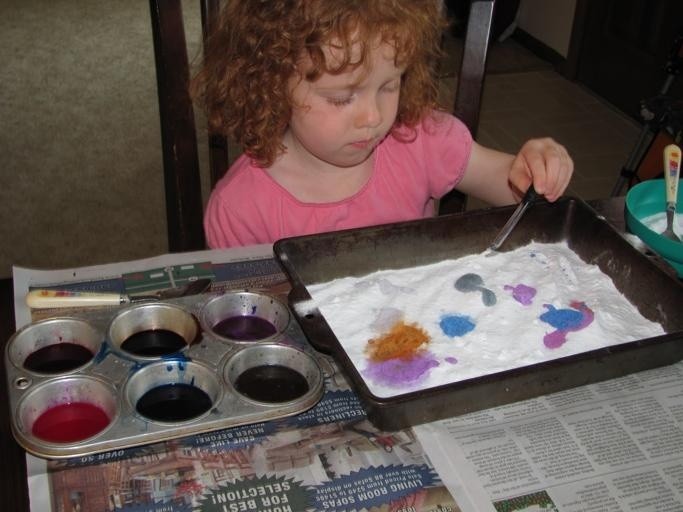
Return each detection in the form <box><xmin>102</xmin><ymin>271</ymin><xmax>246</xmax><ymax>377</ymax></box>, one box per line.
<box><xmin>624</xmin><ymin>177</ymin><xmax>683</xmax><ymax>282</ymax></box>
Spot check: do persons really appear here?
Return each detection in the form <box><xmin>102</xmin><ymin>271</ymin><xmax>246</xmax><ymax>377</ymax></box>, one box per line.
<box><xmin>186</xmin><ymin>1</ymin><xmax>575</xmax><ymax>251</ymax></box>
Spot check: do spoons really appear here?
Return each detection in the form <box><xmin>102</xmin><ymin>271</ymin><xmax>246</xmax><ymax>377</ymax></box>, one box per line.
<box><xmin>658</xmin><ymin>143</ymin><xmax>682</xmax><ymax>243</ymax></box>
<box><xmin>25</xmin><ymin>278</ymin><xmax>213</xmax><ymax>309</ymax></box>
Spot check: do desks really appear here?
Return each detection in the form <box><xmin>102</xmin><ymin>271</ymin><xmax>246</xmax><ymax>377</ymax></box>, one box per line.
<box><xmin>4</xmin><ymin>199</ymin><xmax>681</xmax><ymax>512</ymax></box>
